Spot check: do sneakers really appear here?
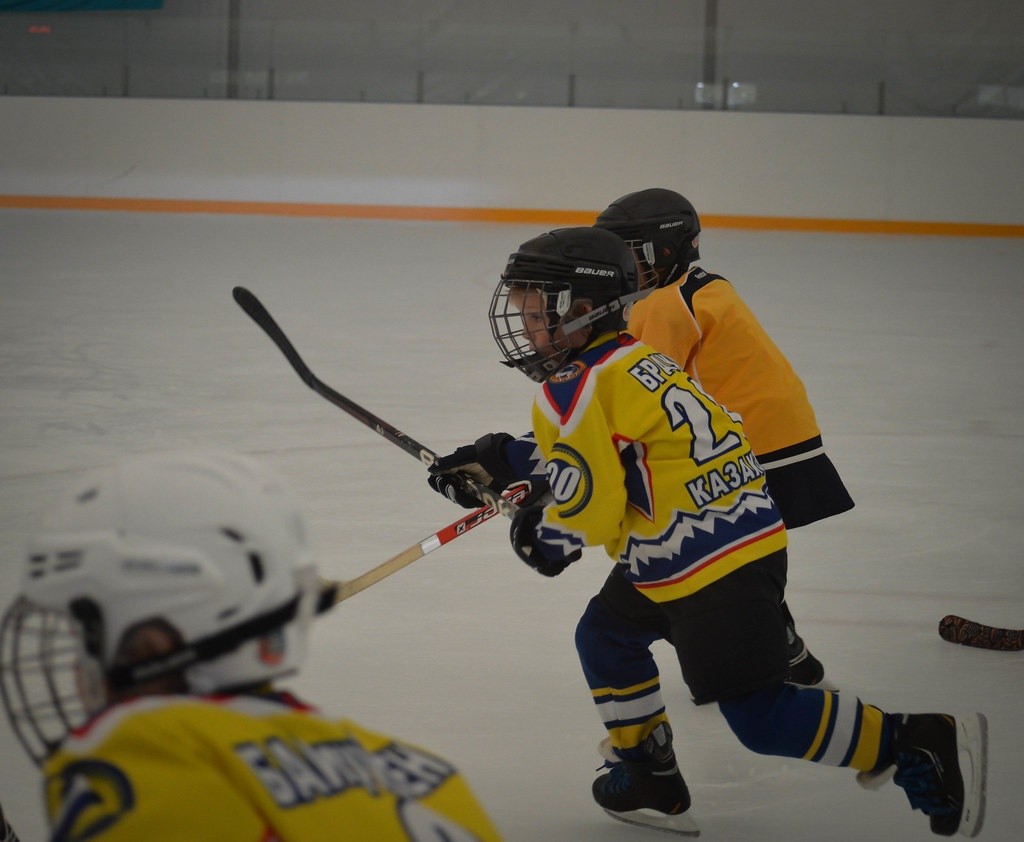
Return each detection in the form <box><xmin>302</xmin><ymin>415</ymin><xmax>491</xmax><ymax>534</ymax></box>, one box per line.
<box><xmin>856</xmin><ymin>712</ymin><xmax>986</xmax><ymax>837</ymax></box>
<box><xmin>592</xmin><ymin>735</ymin><xmax>699</xmax><ymax>834</ymax></box>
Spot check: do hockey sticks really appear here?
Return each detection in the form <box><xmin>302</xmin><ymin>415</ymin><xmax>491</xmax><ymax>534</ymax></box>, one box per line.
<box><xmin>230</xmin><ymin>284</ymin><xmax>520</xmax><ymax>522</ymax></box>
<box><xmin>938</xmin><ymin>612</ymin><xmax>1024</xmax><ymax>651</ymax></box>
<box><xmin>339</xmin><ymin>484</ymin><xmax>525</xmax><ymax>608</ymax></box>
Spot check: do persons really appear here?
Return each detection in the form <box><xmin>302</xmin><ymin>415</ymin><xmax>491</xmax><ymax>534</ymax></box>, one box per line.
<box><xmin>426</xmin><ymin>227</ymin><xmax>990</xmax><ymax>841</ymax></box>
<box><xmin>0</xmin><ymin>458</ymin><xmax>501</xmax><ymax>842</ymax></box>
<box><xmin>596</xmin><ymin>186</ymin><xmax>898</xmax><ymax>791</ymax></box>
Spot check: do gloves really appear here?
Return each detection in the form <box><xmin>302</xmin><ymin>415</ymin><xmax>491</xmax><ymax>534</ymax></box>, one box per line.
<box><xmin>510</xmin><ymin>502</ymin><xmax>581</xmax><ymax>577</ymax></box>
<box><xmin>426</xmin><ymin>430</ymin><xmax>547</xmax><ymax>508</ymax></box>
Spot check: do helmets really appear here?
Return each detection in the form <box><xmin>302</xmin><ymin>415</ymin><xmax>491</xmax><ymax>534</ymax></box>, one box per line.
<box><xmin>594</xmin><ymin>188</ymin><xmax>700</xmax><ymax>291</ymax></box>
<box><xmin>488</xmin><ymin>225</ymin><xmax>656</xmax><ymax>383</ymax></box>
<box><xmin>0</xmin><ymin>457</ymin><xmax>298</xmax><ymax>772</ymax></box>
